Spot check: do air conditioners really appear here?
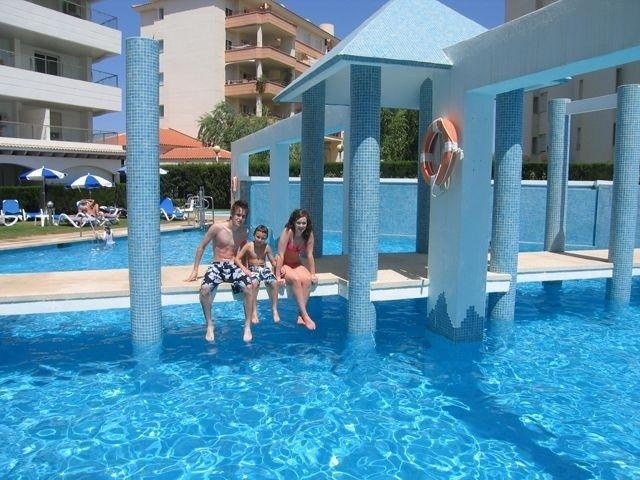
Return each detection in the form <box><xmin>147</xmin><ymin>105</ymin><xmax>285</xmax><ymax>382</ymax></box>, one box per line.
<box><xmin>63</xmin><ymin>2</ymin><xmax>81</xmax><ymax>16</ymax></box>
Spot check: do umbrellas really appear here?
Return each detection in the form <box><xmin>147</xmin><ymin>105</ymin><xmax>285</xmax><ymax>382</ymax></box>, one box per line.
<box><xmin>18</xmin><ymin>164</ymin><xmax>69</xmax><ymax>215</ymax></box>
<box><xmin>117</xmin><ymin>164</ymin><xmax>168</xmax><ymax>176</ymax></box>
<box><xmin>64</xmin><ymin>172</ymin><xmax>114</xmax><ymax>201</ymax></box>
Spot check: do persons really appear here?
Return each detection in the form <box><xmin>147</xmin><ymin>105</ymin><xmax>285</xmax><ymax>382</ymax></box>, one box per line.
<box><xmin>100</xmin><ymin>226</ymin><xmax>115</xmax><ymax>246</ymax></box>
<box><xmin>187</xmin><ymin>200</ymin><xmax>253</xmax><ymax>342</ymax></box>
<box><xmin>235</xmin><ymin>225</ymin><xmax>285</xmax><ymax>325</ymax></box>
<box><xmin>276</xmin><ymin>209</ymin><xmax>318</xmax><ymax>331</ymax></box>
<box><xmin>79</xmin><ymin>199</ymin><xmax>121</xmax><ymax>218</ymax></box>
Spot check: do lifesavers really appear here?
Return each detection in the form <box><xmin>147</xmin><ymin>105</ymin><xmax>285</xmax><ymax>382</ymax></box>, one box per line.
<box><xmin>418</xmin><ymin>115</ymin><xmax>458</xmax><ymax>191</ymax></box>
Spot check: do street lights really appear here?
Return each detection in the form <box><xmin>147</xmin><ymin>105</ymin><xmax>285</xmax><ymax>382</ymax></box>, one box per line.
<box><xmin>213</xmin><ymin>146</ymin><xmax>221</xmax><ymax>163</ymax></box>
<box><xmin>336</xmin><ymin>143</ymin><xmax>343</xmax><ymax>162</ymax></box>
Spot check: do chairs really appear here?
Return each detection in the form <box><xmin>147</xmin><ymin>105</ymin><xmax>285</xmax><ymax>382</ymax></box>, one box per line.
<box><xmin>0</xmin><ymin>197</ymin><xmax>194</xmax><ymax>227</ymax></box>
